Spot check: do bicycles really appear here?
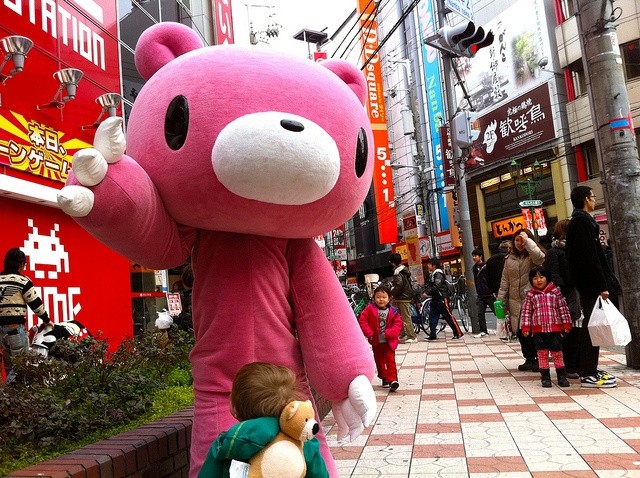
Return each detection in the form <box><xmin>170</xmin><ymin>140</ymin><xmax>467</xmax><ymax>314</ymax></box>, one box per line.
<box><xmin>343</xmin><ymin>285</ymin><xmax>372</xmax><ymax>318</ymax></box>
<box><xmin>419</xmin><ymin>275</ymin><xmax>469</xmax><ymax>336</ymax></box>
<box><xmin>409</xmin><ymin>284</ymin><xmax>446</xmax><ymax>333</ymax></box>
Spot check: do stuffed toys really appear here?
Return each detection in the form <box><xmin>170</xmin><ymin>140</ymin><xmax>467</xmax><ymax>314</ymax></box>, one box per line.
<box><xmin>55</xmin><ymin>19</ymin><xmax>378</xmax><ymax>477</ymax></box>
<box><xmin>246</xmin><ymin>398</ymin><xmax>320</xmax><ymax>477</ymax></box>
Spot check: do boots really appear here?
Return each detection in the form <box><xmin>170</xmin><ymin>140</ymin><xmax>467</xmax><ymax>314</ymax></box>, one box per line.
<box><xmin>540</xmin><ymin>368</ymin><xmax>551</xmax><ymax>386</ymax></box>
<box><xmin>556</xmin><ymin>368</ymin><xmax>569</xmax><ymax>387</ymax></box>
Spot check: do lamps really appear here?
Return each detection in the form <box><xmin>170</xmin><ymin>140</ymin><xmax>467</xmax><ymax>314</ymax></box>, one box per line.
<box><xmin>1</xmin><ymin>35</ymin><xmax>34</xmax><ymax>85</ymax></box>
<box><xmin>537</xmin><ymin>57</ymin><xmax>563</xmax><ymax>77</ymax></box>
<box><xmin>94</xmin><ymin>93</ymin><xmax>121</xmax><ymax>117</ymax></box>
<box><xmin>52</xmin><ymin>67</ymin><xmax>84</xmax><ymax>108</ymax></box>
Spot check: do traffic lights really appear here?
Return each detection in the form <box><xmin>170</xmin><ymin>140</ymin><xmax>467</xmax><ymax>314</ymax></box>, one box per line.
<box><xmin>437</xmin><ymin>20</ymin><xmax>494</xmax><ymax>58</ymax></box>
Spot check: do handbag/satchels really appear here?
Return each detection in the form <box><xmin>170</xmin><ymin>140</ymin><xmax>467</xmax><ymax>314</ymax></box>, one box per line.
<box><xmin>404</xmin><ymin>272</ymin><xmax>428</xmax><ymax>302</ymax></box>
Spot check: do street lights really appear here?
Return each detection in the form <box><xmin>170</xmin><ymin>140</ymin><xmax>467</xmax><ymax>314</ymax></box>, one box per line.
<box><xmin>391</xmin><ymin>162</ymin><xmax>435</xmax><ymax>259</ymax></box>
<box><xmin>510</xmin><ymin>159</ymin><xmax>543</xmax><ymax>241</ymax></box>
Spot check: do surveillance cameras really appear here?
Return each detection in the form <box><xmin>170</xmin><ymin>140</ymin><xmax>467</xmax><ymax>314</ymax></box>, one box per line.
<box><xmin>476</xmin><ymin>157</ymin><xmax>485</xmax><ymax>166</ymax></box>
<box><xmin>418</xmin><ymin>220</ymin><xmax>426</xmax><ymax>226</ymax></box>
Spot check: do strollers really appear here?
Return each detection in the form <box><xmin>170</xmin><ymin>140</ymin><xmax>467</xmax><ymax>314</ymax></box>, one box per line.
<box><xmin>28</xmin><ymin>320</ymin><xmax>102</xmax><ymax>385</ymax></box>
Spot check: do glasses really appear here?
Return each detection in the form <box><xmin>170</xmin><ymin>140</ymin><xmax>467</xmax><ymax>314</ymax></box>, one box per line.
<box><xmin>588</xmin><ymin>195</ymin><xmax>596</xmax><ymax>198</ymax></box>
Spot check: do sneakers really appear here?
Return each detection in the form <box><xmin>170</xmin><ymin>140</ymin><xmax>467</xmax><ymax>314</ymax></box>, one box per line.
<box><xmin>382</xmin><ymin>378</ymin><xmax>389</xmax><ymax>388</ymax></box>
<box><xmin>424</xmin><ymin>337</ymin><xmax>437</xmax><ymax>341</ymax></box>
<box><xmin>474</xmin><ymin>332</ymin><xmax>489</xmax><ymax>338</ymax></box>
<box><xmin>388</xmin><ymin>380</ymin><xmax>399</xmax><ymax>392</ymax></box>
<box><xmin>580</xmin><ymin>375</ymin><xmax>617</xmax><ymax>388</ymax></box>
<box><xmin>597</xmin><ymin>369</ymin><xmax>616</xmax><ymax>382</ymax></box>
<box><xmin>452</xmin><ymin>333</ymin><xmax>465</xmax><ymax>339</ymax></box>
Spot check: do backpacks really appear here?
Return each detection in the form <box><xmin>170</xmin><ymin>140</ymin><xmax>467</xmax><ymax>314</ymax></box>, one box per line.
<box><xmin>440</xmin><ymin>273</ymin><xmax>455</xmax><ymax>296</ymax></box>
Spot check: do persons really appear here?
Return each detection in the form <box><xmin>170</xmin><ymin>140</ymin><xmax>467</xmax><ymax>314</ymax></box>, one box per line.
<box><xmin>358</xmin><ymin>286</ymin><xmax>402</xmax><ymax>390</ymax></box>
<box><xmin>522</xmin><ymin>265</ymin><xmax>573</xmax><ymax>389</ymax></box>
<box><xmin>494</xmin><ymin>228</ymin><xmax>545</xmax><ymax>373</ymax></box>
<box><xmin>544</xmin><ymin>219</ymin><xmax>578</xmax><ymax>373</ymax></box>
<box><xmin>388</xmin><ymin>250</ymin><xmax>418</xmax><ymax>344</ymax></box>
<box><xmin>0</xmin><ymin>246</ymin><xmax>56</xmax><ymax>383</ymax></box>
<box><xmin>472</xmin><ymin>247</ymin><xmax>500</xmax><ymax>342</ymax></box>
<box><xmin>566</xmin><ymin>185</ymin><xmax>621</xmax><ymax>391</ymax></box>
<box><xmin>421</xmin><ymin>256</ymin><xmax>466</xmax><ymax>343</ymax></box>
<box><xmin>197</xmin><ymin>361</ymin><xmax>348</xmax><ymax>477</ymax></box>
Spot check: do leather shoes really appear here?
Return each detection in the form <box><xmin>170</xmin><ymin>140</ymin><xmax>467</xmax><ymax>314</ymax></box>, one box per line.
<box><xmin>566</xmin><ymin>372</ymin><xmax>579</xmax><ymax>378</ymax></box>
<box><xmin>518</xmin><ymin>358</ymin><xmax>540</xmax><ymax>372</ymax></box>
